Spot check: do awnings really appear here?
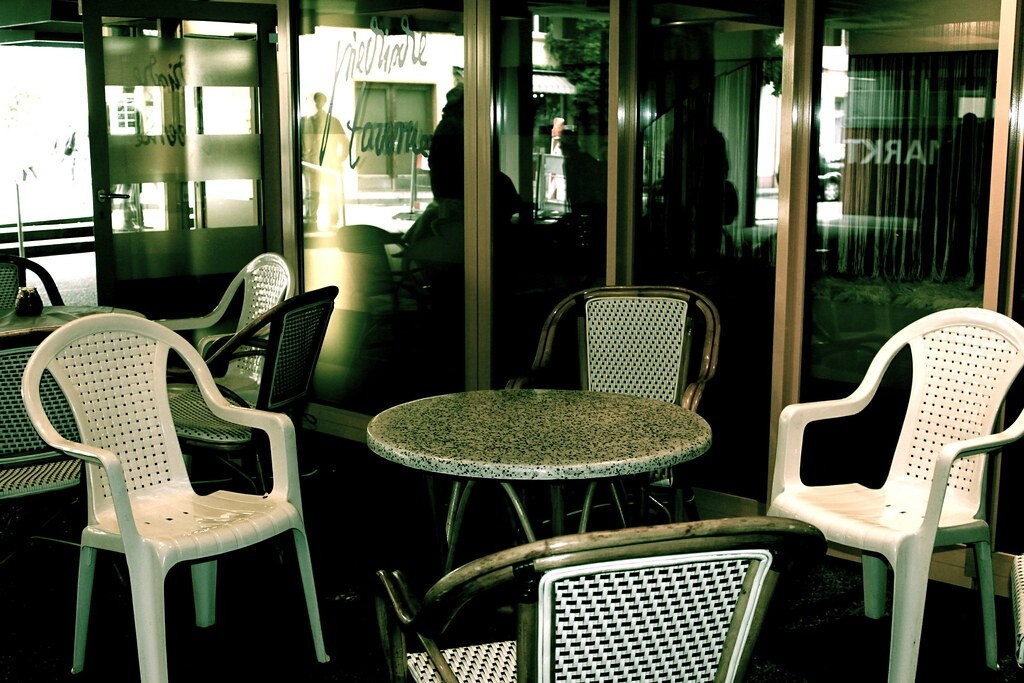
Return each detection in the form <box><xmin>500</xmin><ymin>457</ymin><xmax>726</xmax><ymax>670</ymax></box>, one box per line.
<box><xmin>453</xmin><ymin>66</ymin><xmax>576</xmax><ymax>95</ymax></box>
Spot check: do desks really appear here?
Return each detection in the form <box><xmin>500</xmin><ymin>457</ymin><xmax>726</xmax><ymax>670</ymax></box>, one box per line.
<box><xmin>365</xmin><ymin>389</ymin><xmax>714</xmax><ymax>633</ymax></box>
<box><xmin>0</xmin><ymin>306</ymin><xmax>146</xmax><ymax>331</ymax></box>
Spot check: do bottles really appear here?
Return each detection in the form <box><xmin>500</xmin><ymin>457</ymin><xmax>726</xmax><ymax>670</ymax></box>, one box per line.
<box><xmin>14</xmin><ymin>286</ymin><xmax>43</xmax><ymax>318</ymax></box>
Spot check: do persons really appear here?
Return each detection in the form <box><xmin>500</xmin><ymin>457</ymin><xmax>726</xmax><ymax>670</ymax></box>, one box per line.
<box><xmin>110</xmin><ymin>84</ymin><xmax>145</xmax><ymax>230</ymax></box>
<box><xmin>429</xmin><ymin>83</ymin><xmax>501</xmax><ymax>202</ymax></box>
<box><xmin>662</xmin><ymin>96</ymin><xmax>729</xmax><ymax>254</ymax></box>
<box><xmin>302</xmin><ymin>92</ymin><xmax>350</xmax><ymax>232</ymax></box>
<box><xmin>559</xmin><ymin>128</ymin><xmax>602</xmax><ymax>215</ymax></box>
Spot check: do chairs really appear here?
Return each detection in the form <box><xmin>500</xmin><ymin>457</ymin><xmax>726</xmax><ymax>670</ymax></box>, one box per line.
<box><xmin>370</xmin><ymin>518</ymin><xmax>831</xmax><ymax>682</ymax></box>
<box><xmin>354</xmin><ymin>227</ymin><xmax>441</xmax><ymax>320</ymax></box>
<box><xmin>21</xmin><ymin>313</ymin><xmax>330</xmax><ymax>683</ymax></box>
<box><xmin>445</xmin><ymin>283</ymin><xmax>723</xmax><ymax>577</ymax></box>
<box><xmin>757</xmin><ymin>307</ymin><xmax>1024</xmax><ymax>683</ymax></box>
<box><xmin>0</xmin><ymin>250</ymin><xmax>338</xmax><ymax>592</ymax></box>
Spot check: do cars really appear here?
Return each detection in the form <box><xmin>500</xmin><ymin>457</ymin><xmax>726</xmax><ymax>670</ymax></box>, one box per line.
<box><xmin>774</xmin><ymin>143</ymin><xmax>845</xmax><ymax>203</ymax></box>
